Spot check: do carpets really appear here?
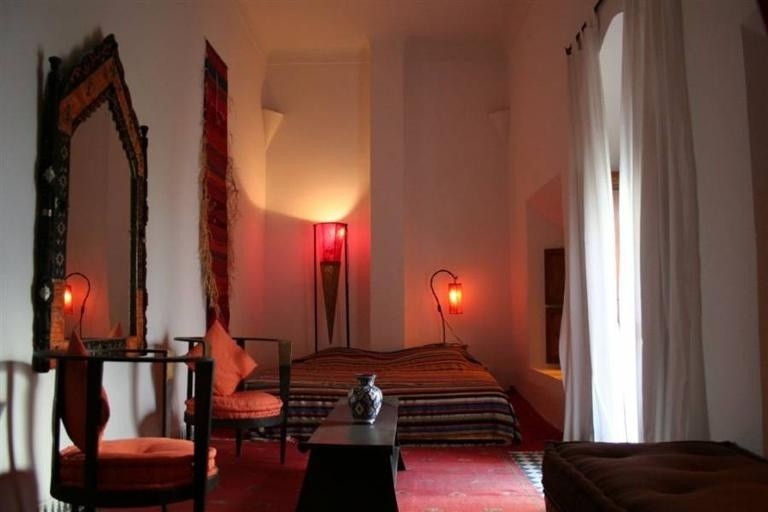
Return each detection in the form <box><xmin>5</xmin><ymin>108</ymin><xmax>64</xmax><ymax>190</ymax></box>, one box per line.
<box><xmin>507</xmin><ymin>450</ymin><xmax>545</xmax><ymax>497</ymax></box>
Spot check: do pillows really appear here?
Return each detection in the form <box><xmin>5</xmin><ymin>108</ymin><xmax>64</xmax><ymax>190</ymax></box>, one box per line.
<box><xmin>57</xmin><ymin>331</ymin><xmax>110</xmax><ymax>455</ymax></box>
<box><xmin>183</xmin><ymin>320</ymin><xmax>259</xmax><ymax>398</ymax></box>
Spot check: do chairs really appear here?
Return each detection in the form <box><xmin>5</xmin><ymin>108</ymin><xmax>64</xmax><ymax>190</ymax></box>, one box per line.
<box><xmin>50</xmin><ymin>348</ymin><xmax>220</xmax><ymax>511</ymax></box>
<box><xmin>173</xmin><ymin>337</ymin><xmax>288</xmax><ymax>465</ymax></box>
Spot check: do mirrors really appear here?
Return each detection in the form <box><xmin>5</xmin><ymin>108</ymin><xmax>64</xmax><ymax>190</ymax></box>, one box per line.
<box><xmin>33</xmin><ymin>32</ymin><xmax>150</xmax><ymax>375</ymax></box>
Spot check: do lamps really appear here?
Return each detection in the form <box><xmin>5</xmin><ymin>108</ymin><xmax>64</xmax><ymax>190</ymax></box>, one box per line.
<box><xmin>429</xmin><ymin>270</ymin><xmax>463</xmax><ymax>345</ymax></box>
<box><xmin>313</xmin><ymin>223</ymin><xmax>350</xmax><ymax>351</ymax></box>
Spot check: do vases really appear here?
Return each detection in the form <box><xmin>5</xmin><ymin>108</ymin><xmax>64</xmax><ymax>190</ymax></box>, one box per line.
<box><xmin>347</xmin><ymin>373</ymin><xmax>384</xmax><ymax>426</ymax></box>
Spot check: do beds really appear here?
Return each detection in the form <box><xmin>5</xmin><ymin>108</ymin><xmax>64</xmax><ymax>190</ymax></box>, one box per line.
<box><xmin>244</xmin><ymin>343</ymin><xmax>521</xmax><ymax>448</ymax></box>
